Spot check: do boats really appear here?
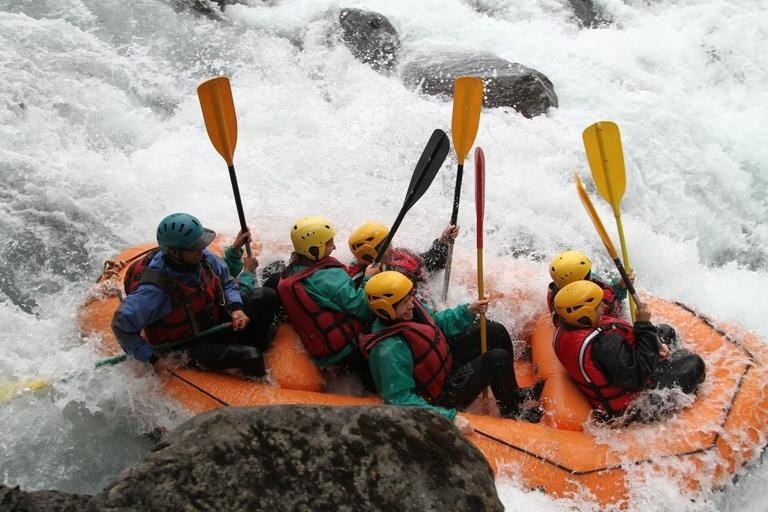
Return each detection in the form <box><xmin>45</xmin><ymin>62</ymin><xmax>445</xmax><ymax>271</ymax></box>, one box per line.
<box><xmin>80</xmin><ymin>231</ymin><xmax>768</xmax><ymax>511</ymax></box>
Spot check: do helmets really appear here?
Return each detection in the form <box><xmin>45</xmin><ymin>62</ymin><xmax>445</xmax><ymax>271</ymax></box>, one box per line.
<box><xmin>549</xmin><ymin>251</ymin><xmax>592</xmax><ymax>288</ymax></box>
<box><xmin>348</xmin><ymin>223</ymin><xmax>388</xmax><ymax>265</ymax></box>
<box><xmin>290</xmin><ymin>216</ymin><xmax>336</xmax><ymax>261</ymax></box>
<box><xmin>364</xmin><ymin>271</ymin><xmax>414</xmax><ymax>320</ymax></box>
<box><xmin>554</xmin><ymin>280</ymin><xmax>604</xmax><ymax>327</ymax></box>
<box><xmin>156</xmin><ymin>213</ymin><xmax>216</xmax><ymax>261</ymax></box>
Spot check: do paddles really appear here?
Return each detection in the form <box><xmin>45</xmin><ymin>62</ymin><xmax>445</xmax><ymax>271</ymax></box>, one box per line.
<box><xmin>372</xmin><ymin>128</ymin><xmax>451</xmax><ymax>269</ymax></box>
<box><xmin>441</xmin><ymin>74</ymin><xmax>484</xmax><ymax>304</ymax></box>
<box><xmin>1</xmin><ymin>319</ymin><xmax>244</xmax><ymax>406</ymax></box>
<box><xmin>196</xmin><ymin>75</ymin><xmax>253</xmax><ymax>259</ymax></box>
<box><xmin>473</xmin><ymin>146</ymin><xmax>489</xmax><ymax>401</ymax></box>
<box><xmin>574</xmin><ymin>172</ymin><xmax>666</xmax><ymax>354</ymax></box>
<box><xmin>581</xmin><ymin>119</ymin><xmax>637</xmax><ymax>326</ymax></box>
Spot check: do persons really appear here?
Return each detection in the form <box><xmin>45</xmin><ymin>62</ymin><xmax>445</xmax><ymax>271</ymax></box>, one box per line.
<box><xmin>361</xmin><ymin>269</ymin><xmax>546</xmax><ymax>424</ymax></box>
<box><xmin>218</xmin><ymin>228</ymin><xmax>259</xmax><ymax>295</ymax></box>
<box><xmin>546</xmin><ymin>250</ymin><xmax>636</xmax><ymax>329</ymax></box>
<box><xmin>275</xmin><ymin>216</ymin><xmax>381</xmax><ymax>394</ymax></box>
<box><xmin>110</xmin><ymin>213</ymin><xmax>282</xmax><ymax>378</ymax></box>
<box><xmin>344</xmin><ymin>220</ymin><xmax>460</xmax><ymax>289</ymax></box>
<box><xmin>551</xmin><ymin>279</ymin><xmax>705</xmax><ymax>428</ymax></box>
